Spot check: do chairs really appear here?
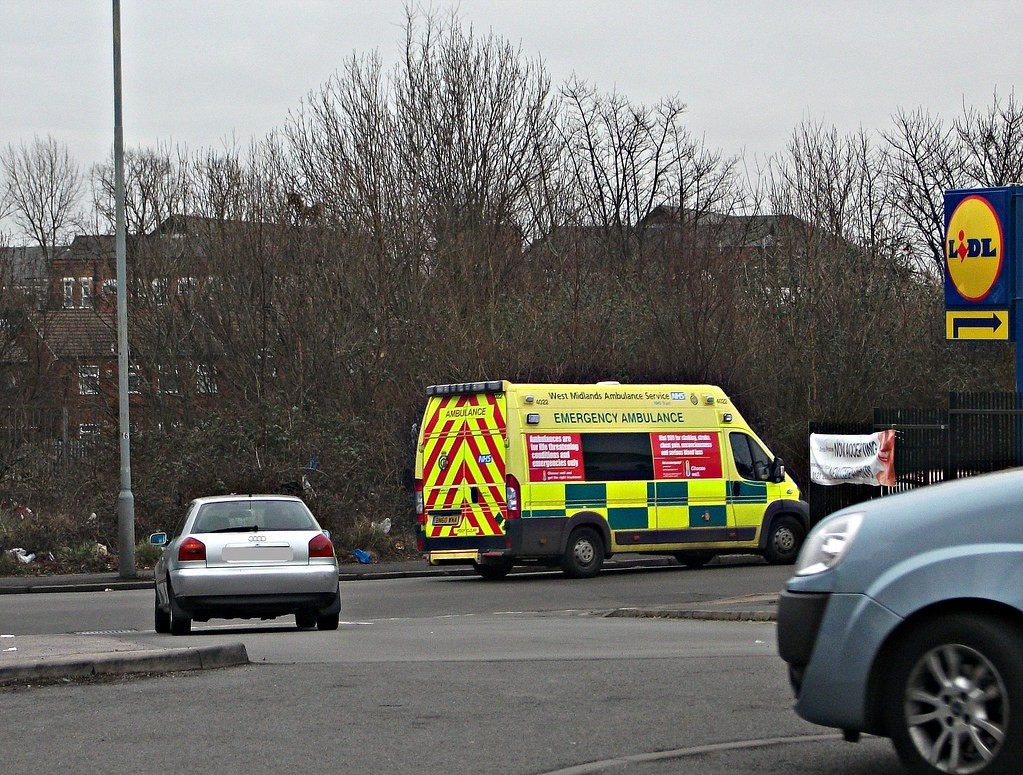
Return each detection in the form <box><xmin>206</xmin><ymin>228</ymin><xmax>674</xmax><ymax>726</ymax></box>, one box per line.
<box><xmin>205</xmin><ymin>516</ymin><xmax>230</xmax><ymax>530</ymax></box>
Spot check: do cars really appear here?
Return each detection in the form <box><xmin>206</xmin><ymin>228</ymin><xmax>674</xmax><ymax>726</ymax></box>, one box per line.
<box><xmin>149</xmin><ymin>487</ymin><xmax>341</xmax><ymax>635</ymax></box>
<box><xmin>776</xmin><ymin>464</ymin><xmax>1023</xmax><ymax>775</ymax></box>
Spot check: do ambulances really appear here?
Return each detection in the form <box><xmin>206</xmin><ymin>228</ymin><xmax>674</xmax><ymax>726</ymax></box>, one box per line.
<box><xmin>413</xmin><ymin>380</ymin><xmax>810</xmax><ymax>580</ymax></box>
